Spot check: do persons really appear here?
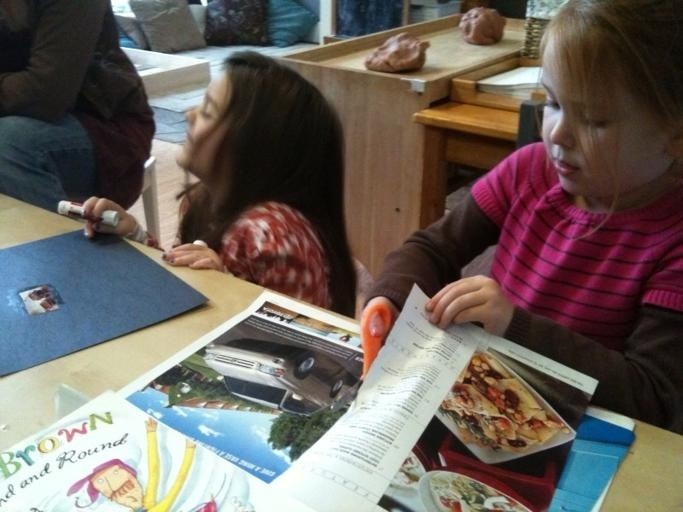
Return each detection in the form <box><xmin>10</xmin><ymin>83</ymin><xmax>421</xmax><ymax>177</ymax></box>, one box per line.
<box><xmin>360</xmin><ymin>2</ymin><xmax>683</xmax><ymax>432</ymax></box>
<box><xmin>0</xmin><ymin>0</ymin><xmax>156</xmax><ymax>223</ymax></box>
<box><xmin>81</xmin><ymin>52</ymin><xmax>356</xmax><ymax>323</ymax></box>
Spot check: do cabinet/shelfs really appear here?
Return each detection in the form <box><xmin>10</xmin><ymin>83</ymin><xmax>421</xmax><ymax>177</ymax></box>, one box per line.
<box><xmin>274</xmin><ymin>12</ymin><xmax>526</xmax><ymax>287</ymax></box>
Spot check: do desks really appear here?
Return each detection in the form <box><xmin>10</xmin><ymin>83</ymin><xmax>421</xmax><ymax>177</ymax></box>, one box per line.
<box><xmin>413</xmin><ymin>102</ymin><xmax>520</xmax><ymax>282</ymax></box>
<box><xmin>0</xmin><ymin>192</ymin><xmax>683</xmax><ymax>512</ymax></box>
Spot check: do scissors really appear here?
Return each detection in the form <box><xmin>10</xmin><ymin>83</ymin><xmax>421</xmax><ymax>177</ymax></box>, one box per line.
<box><xmin>353</xmin><ymin>296</ymin><xmax>396</xmax><ymax>401</ymax></box>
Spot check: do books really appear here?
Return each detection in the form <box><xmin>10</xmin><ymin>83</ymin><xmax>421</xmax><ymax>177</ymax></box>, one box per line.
<box><xmin>476</xmin><ymin>66</ymin><xmax>547</xmax><ymax>102</ymax></box>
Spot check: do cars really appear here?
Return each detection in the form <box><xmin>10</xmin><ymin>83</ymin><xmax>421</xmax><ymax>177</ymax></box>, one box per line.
<box><xmin>201</xmin><ymin>340</ymin><xmax>342</xmax><ymax>416</ymax></box>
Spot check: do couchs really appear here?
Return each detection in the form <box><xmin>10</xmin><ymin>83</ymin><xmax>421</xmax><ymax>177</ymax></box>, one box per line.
<box><xmin>109</xmin><ymin>10</ymin><xmax>321</xmax><ymax>144</ymax></box>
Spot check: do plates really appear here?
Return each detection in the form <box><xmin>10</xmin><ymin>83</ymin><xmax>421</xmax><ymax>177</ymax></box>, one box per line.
<box><xmin>431</xmin><ymin>344</ymin><xmax>577</xmax><ymax>464</ymax></box>
<box><xmin>388</xmin><ymin>447</ymin><xmax>544</xmax><ymax>512</ymax></box>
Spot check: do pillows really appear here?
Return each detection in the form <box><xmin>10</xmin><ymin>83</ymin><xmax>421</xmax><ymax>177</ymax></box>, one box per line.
<box><xmin>113</xmin><ymin>13</ymin><xmax>147</xmax><ymax>49</ymax></box>
<box><xmin>127</xmin><ymin>1</ymin><xmax>206</xmax><ymax>53</ymax></box>
<box><xmin>267</xmin><ymin>1</ymin><xmax>320</xmax><ymax>49</ymax></box>
<box><xmin>205</xmin><ymin>0</ymin><xmax>271</xmax><ymax>46</ymax></box>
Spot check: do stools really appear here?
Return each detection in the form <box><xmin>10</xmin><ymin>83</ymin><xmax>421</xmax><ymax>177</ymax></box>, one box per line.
<box><xmin>140</xmin><ymin>157</ymin><xmax>162</xmax><ymax>242</ymax></box>
